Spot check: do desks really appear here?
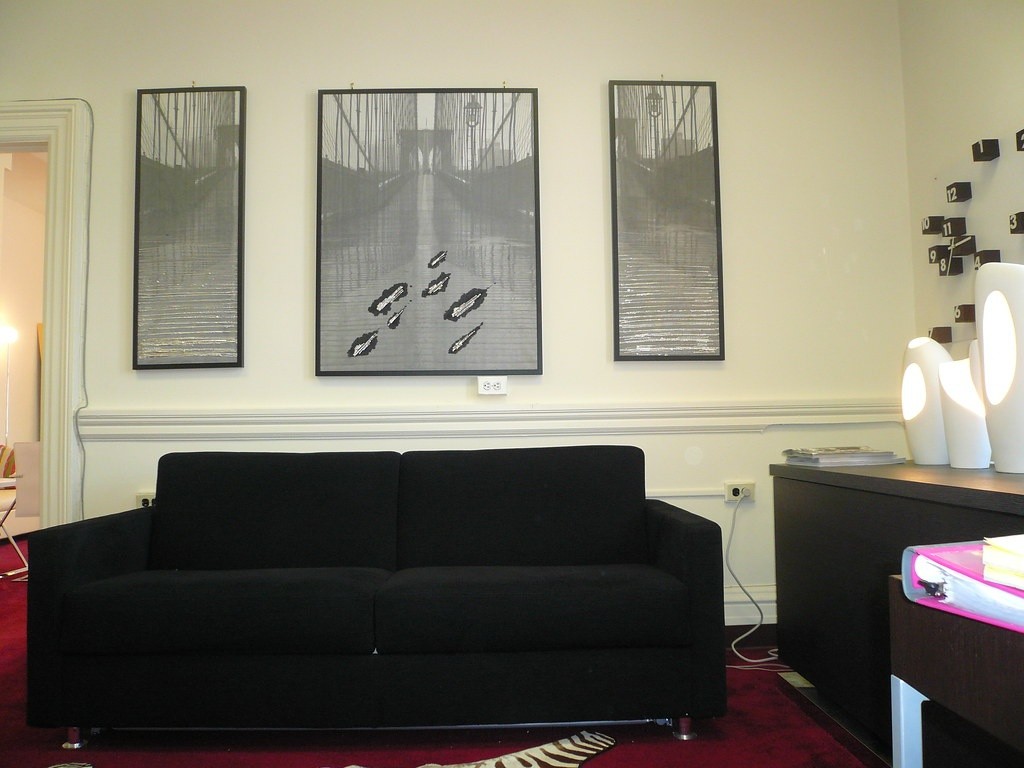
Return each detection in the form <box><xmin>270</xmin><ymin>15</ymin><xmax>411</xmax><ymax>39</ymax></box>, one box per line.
<box><xmin>769</xmin><ymin>460</ymin><xmax>1024</xmax><ymax>761</ymax></box>
<box><xmin>0</xmin><ymin>445</ymin><xmax>29</xmax><ymax>582</ymax></box>
<box><xmin>888</xmin><ymin>574</ymin><xmax>1024</xmax><ymax>768</ymax></box>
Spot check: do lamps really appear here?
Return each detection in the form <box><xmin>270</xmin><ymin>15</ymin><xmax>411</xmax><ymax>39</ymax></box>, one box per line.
<box><xmin>901</xmin><ymin>263</ymin><xmax>1024</xmax><ymax>473</ymax></box>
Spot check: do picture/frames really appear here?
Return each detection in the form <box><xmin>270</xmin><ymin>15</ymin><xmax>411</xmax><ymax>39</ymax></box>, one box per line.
<box><xmin>609</xmin><ymin>80</ymin><xmax>726</xmax><ymax>363</ymax></box>
<box><xmin>315</xmin><ymin>89</ymin><xmax>544</xmax><ymax>376</ymax></box>
<box><xmin>133</xmin><ymin>86</ymin><xmax>246</xmax><ymax>370</ymax></box>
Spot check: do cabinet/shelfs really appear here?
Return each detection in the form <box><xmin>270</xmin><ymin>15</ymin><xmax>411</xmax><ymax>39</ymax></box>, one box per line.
<box><xmin>14</xmin><ymin>441</ymin><xmax>43</xmax><ymax>518</ymax></box>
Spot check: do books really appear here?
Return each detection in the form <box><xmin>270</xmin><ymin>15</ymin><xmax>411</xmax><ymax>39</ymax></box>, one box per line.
<box><xmin>781</xmin><ymin>445</ymin><xmax>906</xmax><ymax>468</ymax></box>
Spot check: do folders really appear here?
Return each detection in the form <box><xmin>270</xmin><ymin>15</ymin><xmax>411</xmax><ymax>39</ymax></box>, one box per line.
<box><xmin>899</xmin><ymin>533</ymin><xmax>1024</xmax><ymax>636</ymax></box>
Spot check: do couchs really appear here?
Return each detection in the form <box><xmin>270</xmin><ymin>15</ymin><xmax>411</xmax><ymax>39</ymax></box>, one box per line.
<box><xmin>28</xmin><ymin>446</ymin><xmax>726</xmax><ymax>749</ymax></box>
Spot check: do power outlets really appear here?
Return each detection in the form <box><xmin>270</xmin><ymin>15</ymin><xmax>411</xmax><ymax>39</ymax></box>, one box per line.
<box><xmin>478</xmin><ymin>375</ymin><xmax>507</xmax><ymax>395</ymax></box>
<box><xmin>723</xmin><ymin>482</ymin><xmax>756</xmax><ymax>504</ymax></box>
<box><xmin>136</xmin><ymin>494</ymin><xmax>156</xmax><ymax>509</ymax></box>
